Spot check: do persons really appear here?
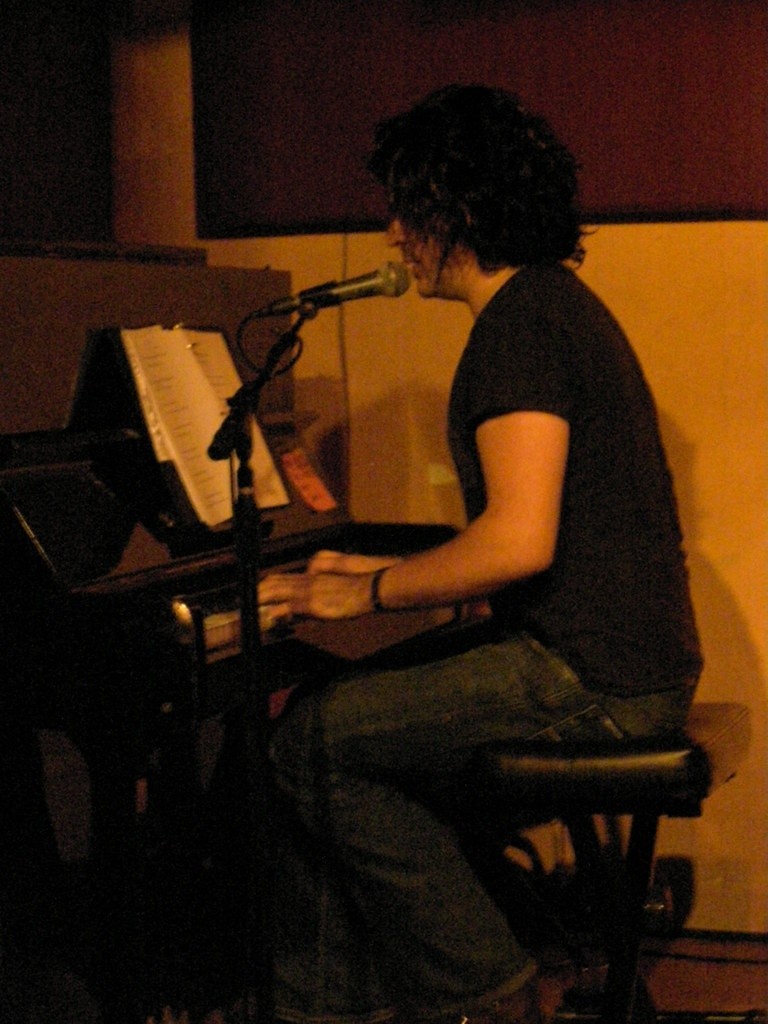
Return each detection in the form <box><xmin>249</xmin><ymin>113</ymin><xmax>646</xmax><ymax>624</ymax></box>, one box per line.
<box><xmin>256</xmin><ymin>81</ymin><xmax>706</xmax><ymax>1024</ymax></box>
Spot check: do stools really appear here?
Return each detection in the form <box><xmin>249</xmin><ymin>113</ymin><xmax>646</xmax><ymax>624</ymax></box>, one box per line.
<box><xmin>467</xmin><ymin>733</ymin><xmax>712</xmax><ymax>1024</ymax></box>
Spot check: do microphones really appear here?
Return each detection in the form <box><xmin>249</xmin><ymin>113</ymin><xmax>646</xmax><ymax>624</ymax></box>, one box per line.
<box><xmin>250</xmin><ymin>261</ymin><xmax>410</xmax><ymax>319</ymax></box>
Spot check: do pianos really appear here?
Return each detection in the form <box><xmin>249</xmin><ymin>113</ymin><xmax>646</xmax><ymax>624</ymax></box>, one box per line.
<box><xmin>0</xmin><ymin>247</ymin><xmax>492</xmax><ymax>1024</ymax></box>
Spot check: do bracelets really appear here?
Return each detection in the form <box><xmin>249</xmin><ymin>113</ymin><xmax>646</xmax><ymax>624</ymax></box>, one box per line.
<box><xmin>368</xmin><ymin>566</ymin><xmax>390</xmax><ymax>615</ymax></box>
